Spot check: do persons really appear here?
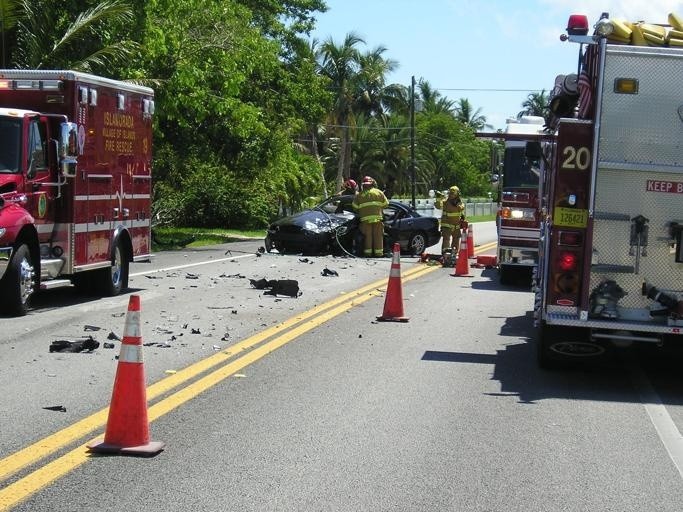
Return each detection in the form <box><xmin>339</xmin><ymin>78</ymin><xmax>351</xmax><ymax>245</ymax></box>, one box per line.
<box><xmin>434</xmin><ymin>186</ymin><xmax>469</xmax><ymax>267</ymax></box>
<box><xmin>352</xmin><ymin>176</ymin><xmax>389</xmax><ymax>257</ymax></box>
<box><xmin>335</xmin><ymin>179</ymin><xmax>360</xmax><ymax>206</ymax></box>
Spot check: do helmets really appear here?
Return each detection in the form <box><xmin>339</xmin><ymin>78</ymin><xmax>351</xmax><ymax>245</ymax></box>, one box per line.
<box><xmin>449</xmin><ymin>185</ymin><xmax>459</xmax><ymax>195</ymax></box>
<box><xmin>362</xmin><ymin>176</ymin><xmax>372</xmax><ymax>185</ymax></box>
<box><xmin>344</xmin><ymin>179</ymin><xmax>357</xmax><ymax>189</ymax></box>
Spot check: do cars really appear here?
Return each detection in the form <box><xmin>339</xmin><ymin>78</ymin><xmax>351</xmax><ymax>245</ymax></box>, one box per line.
<box><xmin>267</xmin><ymin>194</ymin><xmax>441</xmax><ymax>260</ymax></box>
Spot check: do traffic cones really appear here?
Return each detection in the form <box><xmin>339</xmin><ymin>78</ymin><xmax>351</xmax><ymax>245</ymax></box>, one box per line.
<box><xmin>372</xmin><ymin>240</ymin><xmax>410</xmax><ymax>325</ymax></box>
<box><xmin>86</xmin><ymin>292</ymin><xmax>166</xmax><ymax>459</ymax></box>
<box><xmin>448</xmin><ymin>227</ymin><xmax>476</xmax><ymax>277</ymax></box>
<box><xmin>464</xmin><ymin>222</ymin><xmax>478</xmax><ymax>259</ymax></box>
<box><xmin>476</xmin><ymin>256</ymin><xmax>496</xmax><ymax>265</ymax></box>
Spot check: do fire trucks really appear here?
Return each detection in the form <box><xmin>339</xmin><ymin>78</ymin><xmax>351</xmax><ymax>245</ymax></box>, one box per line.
<box><xmin>494</xmin><ymin>113</ymin><xmax>551</xmax><ymax>281</ymax></box>
<box><xmin>0</xmin><ymin>64</ymin><xmax>161</xmax><ymax>318</ymax></box>
<box><xmin>475</xmin><ymin>12</ymin><xmax>683</xmax><ymax>373</ymax></box>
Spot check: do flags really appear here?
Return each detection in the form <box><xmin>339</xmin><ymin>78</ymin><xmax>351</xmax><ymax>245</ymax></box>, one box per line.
<box><xmin>577</xmin><ymin>63</ymin><xmax>592</xmax><ymax>119</ymax></box>
